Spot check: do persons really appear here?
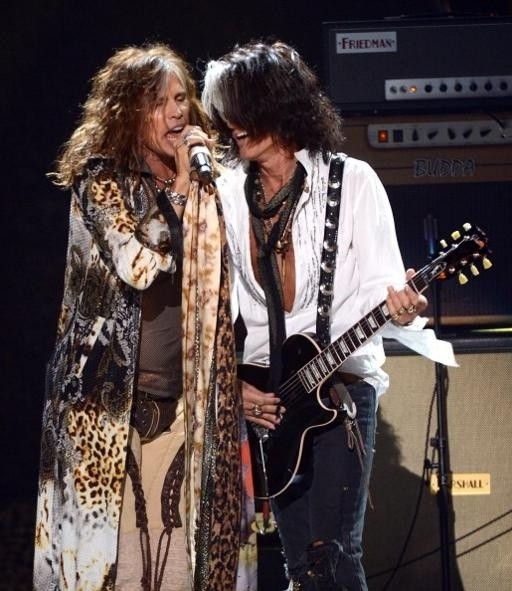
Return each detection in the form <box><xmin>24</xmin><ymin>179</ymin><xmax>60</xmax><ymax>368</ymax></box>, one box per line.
<box><xmin>31</xmin><ymin>38</ymin><xmax>259</xmax><ymax>590</ymax></box>
<box><xmin>197</xmin><ymin>38</ymin><xmax>458</xmax><ymax>590</ymax></box>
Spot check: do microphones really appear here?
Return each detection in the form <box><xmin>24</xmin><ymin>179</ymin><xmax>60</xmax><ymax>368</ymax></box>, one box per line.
<box><xmin>188</xmin><ymin>143</ymin><xmax>213</xmax><ymax>180</ymax></box>
<box><xmin>421</xmin><ymin>211</ymin><xmax>439</xmax><ymax>260</ymax></box>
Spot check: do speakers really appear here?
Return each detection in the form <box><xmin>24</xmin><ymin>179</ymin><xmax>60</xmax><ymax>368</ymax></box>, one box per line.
<box><xmin>319</xmin><ymin>13</ymin><xmax>512</xmax><ymax>113</ymax></box>
<box><xmin>333</xmin><ymin>113</ymin><xmax>512</xmax><ymax>327</ymax></box>
<box><xmin>361</xmin><ymin>333</ymin><xmax>512</xmax><ymax>589</ymax></box>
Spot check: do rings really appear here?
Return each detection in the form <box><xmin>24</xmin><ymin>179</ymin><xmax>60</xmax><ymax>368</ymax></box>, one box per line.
<box><xmin>405</xmin><ymin>302</ymin><xmax>416</xmax><ymax>313</ymax></box>
<box><xmin>392</xmin><ymin>312</ymin><xmax>400</xmax><ymax>320</ymax></box>
<box><xmin>183</xmin><ymin>140</ymin><xmax>190</xmax><ymax>147</ymax></box>
<box><xmin>397</xmin><ymin>306</ymin><xmax>406</xmax><ymax>314</ymax></box>
<box><xmin>251</xmin><ymin>403</ymin><xmax>263</xmax><ymax>416</ymax></box>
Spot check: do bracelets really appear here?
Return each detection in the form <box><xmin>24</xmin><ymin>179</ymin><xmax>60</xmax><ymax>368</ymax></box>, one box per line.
<box><xmin>163</xmin><ymin>186</ymin><xmax>188</xmax><ymax>208</ymax></box>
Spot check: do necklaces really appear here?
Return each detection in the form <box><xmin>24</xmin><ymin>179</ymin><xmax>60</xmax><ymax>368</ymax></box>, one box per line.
<box><xmin>257</xmin><ymin>167</ymin><xmax>309</xmax><ymax>286</ymax></box>
<box><xmin>151</xmin><ymin>166</ymin><xmax>178</xmax><ymax>192</ymax></box>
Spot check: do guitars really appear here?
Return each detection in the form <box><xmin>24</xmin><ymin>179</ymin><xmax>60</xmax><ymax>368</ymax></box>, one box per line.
<box><xmin>237</xmin><ymin>222</ymin><xmax>492</xmax><ymax>500</ymax></box>
